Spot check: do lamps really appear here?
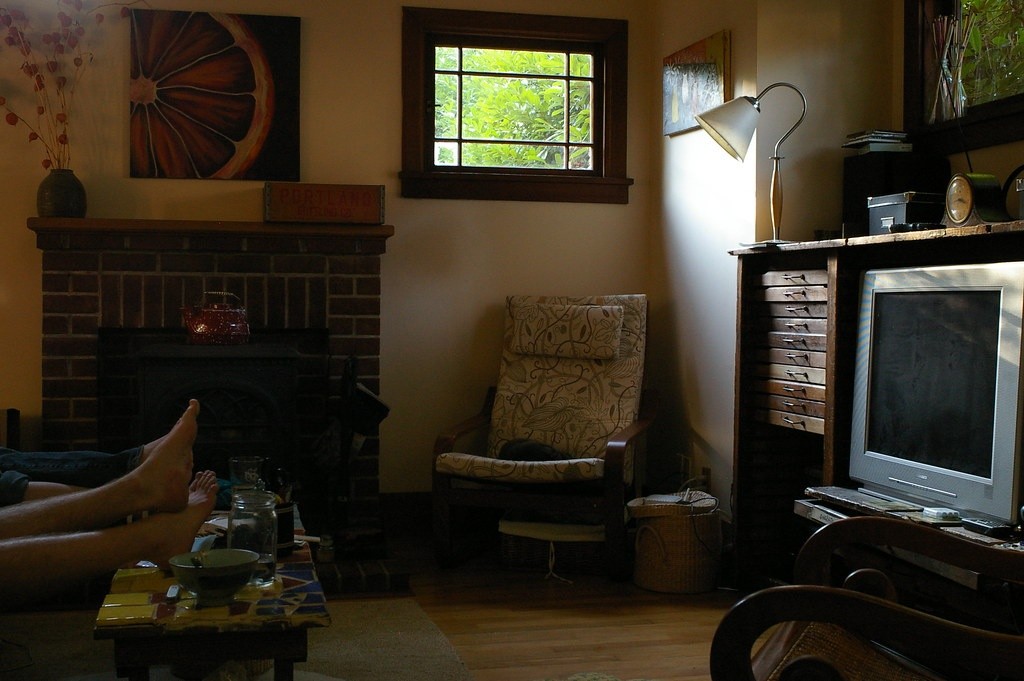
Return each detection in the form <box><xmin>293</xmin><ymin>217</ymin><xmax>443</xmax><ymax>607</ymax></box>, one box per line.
<box><xmin>694</xmin><ymin>81</ymin><xmax>808</xmax><ymax>249</ymax></box>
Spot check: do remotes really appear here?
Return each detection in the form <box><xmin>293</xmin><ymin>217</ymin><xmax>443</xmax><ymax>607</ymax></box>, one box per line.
<box><xmin>891</xmin><ymin>221</ymin><xmax>948</xmax><ymax>233</ymax></box>
<box><xmin>960</xmin><ymin>516</ymin><xmax>1011</xmax><ymax>538</ymax></box>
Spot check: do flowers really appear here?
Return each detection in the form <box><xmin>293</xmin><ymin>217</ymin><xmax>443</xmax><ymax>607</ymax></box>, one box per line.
<box><xmin>0</xmin><ymin>0</ymin><xmax>150</xmax><ymax>177</ymax></box>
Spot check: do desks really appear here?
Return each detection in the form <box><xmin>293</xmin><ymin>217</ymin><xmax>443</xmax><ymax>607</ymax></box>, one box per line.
<box><xmin>94</xmin><ymin>499</ymin><xmax>332</xmax><ymax>681</ymax></box>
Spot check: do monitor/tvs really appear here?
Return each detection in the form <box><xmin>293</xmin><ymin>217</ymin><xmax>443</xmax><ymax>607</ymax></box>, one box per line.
<box><xmin>849</xmin><ymin>262</ymin><xmax>1024</xmax><ymax>528</ymax></box>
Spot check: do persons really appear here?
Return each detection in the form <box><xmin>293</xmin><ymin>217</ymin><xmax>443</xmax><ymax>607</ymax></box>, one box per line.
<box><xmin>0</xmin><ymin>400</ymin><xmax>220</xmax><ymax>588</ymax></box>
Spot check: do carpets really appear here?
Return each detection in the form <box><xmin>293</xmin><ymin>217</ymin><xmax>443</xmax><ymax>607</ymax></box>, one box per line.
<box><xmin>0</xmin><ymin>559</ymin><xmax>474</xmax><ymax>681</ymax></box>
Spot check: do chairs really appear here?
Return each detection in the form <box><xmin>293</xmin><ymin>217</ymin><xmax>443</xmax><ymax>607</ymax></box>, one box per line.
<box><xmin>711</xmin><ymin>516</ymin><xmax>1024</xmax><ymax>681</ymax></box>
<box><xmin>431</xmin><ymin>292</ymin><xmax>671</xmax><ymax>581</ymax></box>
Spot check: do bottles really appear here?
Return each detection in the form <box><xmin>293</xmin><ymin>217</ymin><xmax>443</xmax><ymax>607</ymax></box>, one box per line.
<box><xmin>227</xmin><ymin>492</ymin><xmax>278</xmax><ymax>589</ymax></box>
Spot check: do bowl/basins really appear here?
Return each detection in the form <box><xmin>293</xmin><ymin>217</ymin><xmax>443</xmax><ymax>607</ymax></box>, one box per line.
<box><xmin>169</xmin><ymin>548</ymin><xmax>259</xmax><ymax>600</ymax></box>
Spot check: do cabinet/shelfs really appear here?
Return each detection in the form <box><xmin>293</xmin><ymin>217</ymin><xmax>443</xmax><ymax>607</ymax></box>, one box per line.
<box><xmin>727</xmin><ymin>239</ymin><xmax>848</xmax><ymax>570</ymax></box>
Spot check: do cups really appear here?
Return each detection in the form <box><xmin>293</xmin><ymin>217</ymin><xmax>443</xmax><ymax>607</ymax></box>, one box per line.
<box><xmin>274</xmin><ymin>503</ymin><xmax>295</xmax><ymax>557</ymax></box>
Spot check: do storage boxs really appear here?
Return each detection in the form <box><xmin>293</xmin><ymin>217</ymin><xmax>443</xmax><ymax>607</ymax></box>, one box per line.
<box><xmin>264</xmin><ymin>181</ymin><xmax>386</xmax><ymax>224</ymax></box>
<box><xmin>867</xmin><ymin>190</ymin><xmax>940</xmax><ymax>236</ymax></box>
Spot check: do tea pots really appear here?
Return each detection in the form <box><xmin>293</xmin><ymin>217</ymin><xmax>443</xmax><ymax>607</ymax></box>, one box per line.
<box><xmin>180</xmin><ymin>291</ymin><xmax>249</xmax><ymax>347</ymax></box>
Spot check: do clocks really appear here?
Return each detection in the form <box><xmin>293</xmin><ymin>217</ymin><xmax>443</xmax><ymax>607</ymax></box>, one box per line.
<box><xmin>942</xmin><ymin>171</ymin><xmax>1008</xmax><ymax>228</ymax></box>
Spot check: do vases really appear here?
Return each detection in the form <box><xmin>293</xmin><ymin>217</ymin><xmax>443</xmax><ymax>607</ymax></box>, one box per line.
<box><xmin>36</xmin><ymin>170</ymin><xmax>87</xmax><ymax>220</ymax></box>
